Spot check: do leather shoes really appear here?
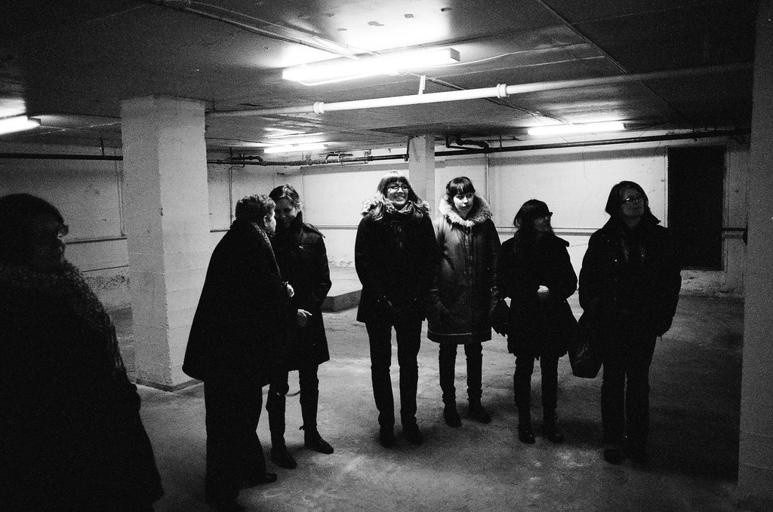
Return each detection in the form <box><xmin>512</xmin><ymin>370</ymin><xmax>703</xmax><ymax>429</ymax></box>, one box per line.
<box><xmin>544</xmin><ymin>423</ymin><xmax>563</xmax><ymax>443</ymax></box>
<box><xmin>519</xmin><ymin>419</ymin><xmax>535</xmax><ymax>444</ymax></box>
<box><xmin>403</xmin><ymin>422</ymin><xmax>425</xmax><ymax>443</ymax></box>
<box><xmin>468</xmin><ymin>402</ymin><xmax>491</xmax><ymax>424</ymax></box>
<box><xmin>379</xmin><ymin>424</ymin><xmax>395</xmax><ymax>448</ymax></box>
<box><xmin>444</xmin><ymin>402</ymin><xmax>461</xmax><ymax>427</ymax></box>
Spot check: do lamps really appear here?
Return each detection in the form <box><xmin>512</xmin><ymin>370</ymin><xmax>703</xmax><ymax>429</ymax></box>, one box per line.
<box><xmin>527</xmin><ymin>122</ymin><xmax>628</xmax><ymax>136</ymax></box>
<box><xmin>264</xmin><ymin>144</ymin><xmax>328</xmax><ymax>153</ymax></box>
<box><xmin>282</xmin><ymin>47</ymin><xmax>460</xmax><ymax>87</ymax></box>
<box><xmin>0</xmin><ymin>115</ymin><xmax>41</xmax><ymax>135</ymax></box>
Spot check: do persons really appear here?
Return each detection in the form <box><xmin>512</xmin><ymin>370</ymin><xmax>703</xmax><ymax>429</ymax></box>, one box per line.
<box><xmin>182</xmin><ymin>192</ymin><xmax>311</xmax><ymax>511</ymax></box>
<box><xmin>492</xmin><ymin>198</ymin><xmax>577</xmax><ymax>444</ymax></box>
<box><xmin>266</xmin><ymin>182</ymin><xmax>333</xmax><ymax>469</ymax></box>
<box><xmin>577</xmin><ymin>179</ymin><xmax>683</xmax><ymax>463</ymax></box>
<box><xmin>0</xmin><ymin>193</ymin><xmax>162</xmax><ymax>509</ymax></box>
<box><xmin>426</xmin><ymin>176</ymin><xmax>502</xmax><ymax>428</ymax></box>
<box><xmin>353</xmin><ymin>170</ymin><xmax>437</xmax><ymax>447</ymax></box>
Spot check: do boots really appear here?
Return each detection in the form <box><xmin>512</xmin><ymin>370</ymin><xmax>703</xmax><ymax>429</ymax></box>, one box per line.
<box><xmin>299</xmin><ymin>388</ymin><xmax>333</xmax><ymax>454</ymax></box>
<box><xmin>266</xmin><ymin>384</ymin><xmax>296</xmax><ymax>469</ymax></box>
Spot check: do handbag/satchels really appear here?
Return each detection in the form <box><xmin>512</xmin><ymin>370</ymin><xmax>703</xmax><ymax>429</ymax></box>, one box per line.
<box><xmin>568</xmin><ymin>312</ymin><xmax>602</xmax><ymax>378</ymax></box>
<box><xmin>491</xmin><ymin>300</ymin><xmax>511</xmax><ymax>337</ymax></box>
